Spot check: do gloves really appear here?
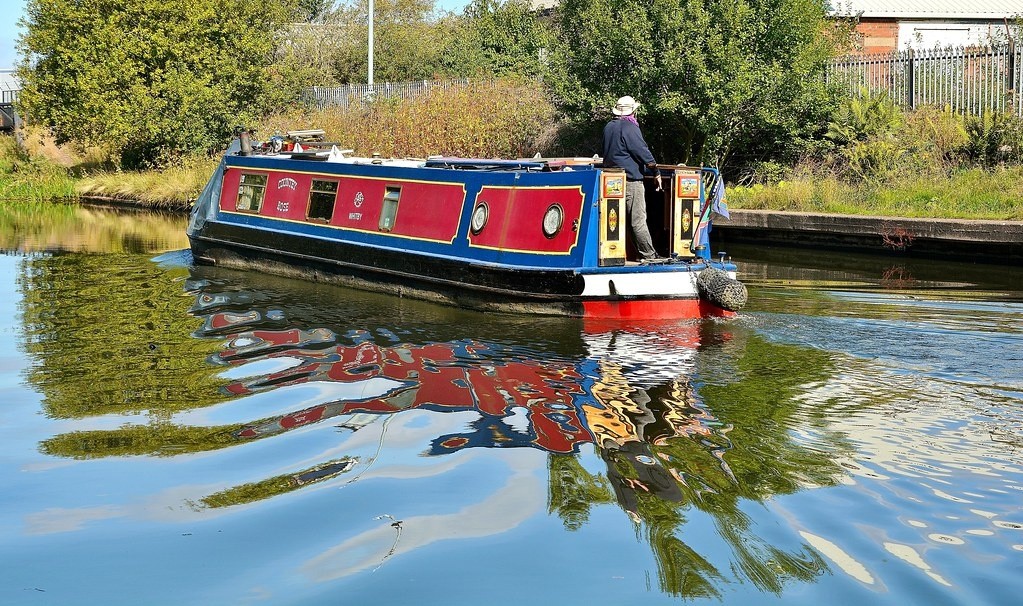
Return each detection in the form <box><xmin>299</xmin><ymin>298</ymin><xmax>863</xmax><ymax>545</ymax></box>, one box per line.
<box><xmin>654</xmin><ymin>175</ymin><xmax>662</xmax><ymax>191</ymax></box>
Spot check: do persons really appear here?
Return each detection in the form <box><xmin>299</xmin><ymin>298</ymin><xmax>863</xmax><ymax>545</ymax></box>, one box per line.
<box><xmin>602</xmin><ymin>95</ymin><xmax>663</xmax><ymax>259</ymax></box>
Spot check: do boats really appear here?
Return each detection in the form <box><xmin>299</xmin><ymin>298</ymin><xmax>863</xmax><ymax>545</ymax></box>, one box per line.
<box><xmin>185</xmin><ymin>126</ymin><xmax>739</xmax><ymax>320</ymax></box>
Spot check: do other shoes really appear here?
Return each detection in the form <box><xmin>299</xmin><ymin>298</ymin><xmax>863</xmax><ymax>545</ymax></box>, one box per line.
<box><xmin>644</xmin><ymin>253</ymin><xmax>667</xmax><ymax>259</ymax></box>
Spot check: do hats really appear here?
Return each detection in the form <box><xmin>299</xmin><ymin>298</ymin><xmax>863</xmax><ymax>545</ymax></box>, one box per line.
<box><xmin>612</xmin><ymin>96</ymin><xmax>640</xmax><ymax>116</ymax></box>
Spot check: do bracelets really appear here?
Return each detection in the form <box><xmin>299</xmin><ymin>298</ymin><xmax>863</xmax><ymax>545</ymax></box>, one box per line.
<box><xmin>655</xmin><ymin>175</ymin><xmax>660</xmax><ymax>179</ymax></box>
